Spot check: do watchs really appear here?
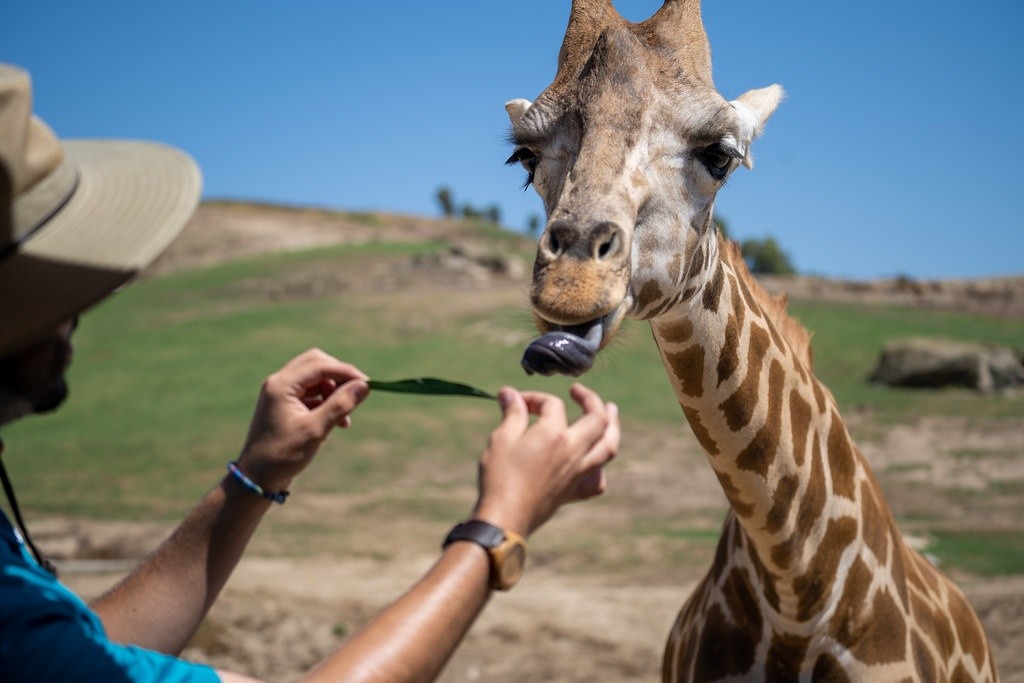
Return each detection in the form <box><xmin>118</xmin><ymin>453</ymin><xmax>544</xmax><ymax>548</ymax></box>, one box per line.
<box><xmin>442</xmin><ymin>519</ymin><xmax>529</xmax><ymax>591</ymax></box>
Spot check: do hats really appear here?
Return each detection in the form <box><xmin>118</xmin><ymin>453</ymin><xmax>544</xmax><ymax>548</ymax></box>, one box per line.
<box><xmin>0</xmin><ymin>63</ymin><xmax>201</xmax><ymax>350</ymax></box>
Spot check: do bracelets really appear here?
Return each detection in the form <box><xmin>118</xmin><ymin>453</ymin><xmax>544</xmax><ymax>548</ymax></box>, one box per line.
<box><xmin>227</xmin><ymin>462</ymin><xmax>285</xmax><ymax>502</ymax></box>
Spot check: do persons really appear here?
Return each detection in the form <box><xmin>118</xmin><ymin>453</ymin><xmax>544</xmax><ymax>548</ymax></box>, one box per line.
<box><xmin>0</xmin><ymin>64</ymin><xmax>622</xmax><ymax>683</ymax></box>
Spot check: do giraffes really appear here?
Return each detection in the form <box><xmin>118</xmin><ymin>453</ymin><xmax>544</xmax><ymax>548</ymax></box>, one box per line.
<box><xmin>506</xmin><ymin>0</ymin><xmax>999</xmax><ymax>683</ymax></box>
